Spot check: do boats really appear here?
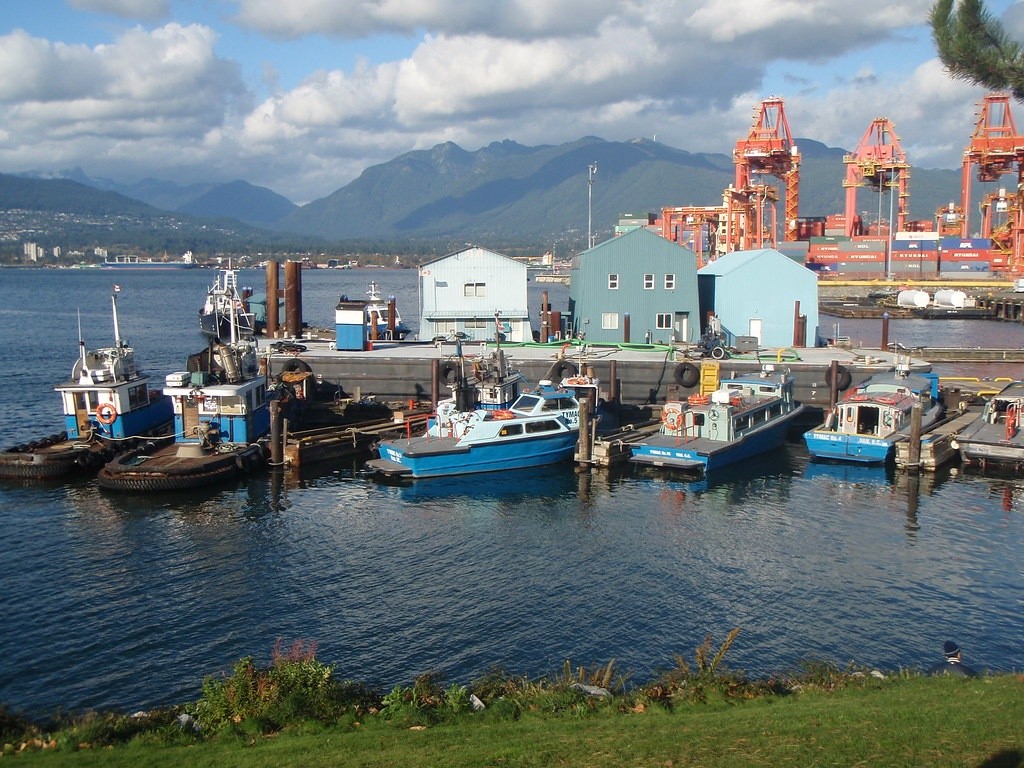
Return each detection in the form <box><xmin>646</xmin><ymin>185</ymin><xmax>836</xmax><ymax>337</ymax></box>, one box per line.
<box><xmin>100</xmin><ymin>250</ymin><xmax>196</xmax><ymax>270</ymax></box>
<box><xmin>197</xmin><ymin>257</ymin><xmax>258</xmax><ymax>339</ymax></box>
<box><xmin>361</xmin><ymin>310</ymin><xmax>604</xmax><ymax>482</ymax></box>
<box><xmin>95</xmin><ymin>254</ymin><xmax>304</xmax><ymax>494</ymax></box>
<box><xmin>800</xmin><ymin>341</ymin><xmax>942</xmax><ymax>464</ymax></box>
<box><xmin>363</xmin><ymin>280</ymin><xmax>412</xmax><ymax>340</ymax></box>
<box><xmin>628</xmin><ymin>357</ymin><xmax>806</xmax><ymax>473</ymax></box>
<box><xmin>0</xmin><ymin>291</ymin><xmax>176</xmax><ymax>483</ymax></box>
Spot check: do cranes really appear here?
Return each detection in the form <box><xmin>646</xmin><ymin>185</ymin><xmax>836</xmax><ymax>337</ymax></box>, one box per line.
<box><xmin>656</xmin><ymin>92</ymin><xmax>1024</xmax><ymax>322</ymax></box>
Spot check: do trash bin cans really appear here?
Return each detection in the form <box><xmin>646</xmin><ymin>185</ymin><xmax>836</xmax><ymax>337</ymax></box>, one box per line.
<box><xmin>736</xmin><ymin>336</ymin><xmax>759</xmax><ymax>355</ymax></box>
<box><xmin>365</xmin><ymin>340</ymin><xmax>373</xmax><ymax>351</ymax></box>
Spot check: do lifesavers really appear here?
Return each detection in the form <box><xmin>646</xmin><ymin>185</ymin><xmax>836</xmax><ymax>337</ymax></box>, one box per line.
<box><xmin>491</xmin><ymin>409</ymin><xmax>514</xmax><ymax>414</ymax></box>
<box><xmin>95</xmin><ymin>403</ymin><xmax>118</xmax><ymax>424</ymax></box>
<box><xmin>282</xmin><ymin>359</ymin><xmax>310</xmax><ymax>373</ymax></box>
<box><xmin>438</xmin><ymin>360</ymin><xmax>460</xmax><ymax>386</ymax></box>
<box><xmin>688</xmin><ymin>394</ymin><xmax>710</xmax><ymax>406</ymax></box>
<box><xmin>875</xmin><ymin>396</ymin><xmax>894</xmax><ymax>404</ymax></box>
<box><xmin>661</xmin><ymin>408</ymin><xmax>684</xmax><ymax>430</ymax></box>
<box><xmin>551</xmin><ymin>360</ymin><xmax>578</xmax><ymax>384</ymax></box>
<box><xmin>849</xmin><ymin>395</ymin><xmax>869</xmax><ymax>401</ymax></box>
<box><xmin>493</xmin><ymin>413</ymin><xmax>516</xmax><ymax>420</ymax></box>
<box><xmin>824</xmin><ymin>365</ymin><xmax>852</xmax><ymax>391</ymax></box>
<box><xmin>673</xmin><ymin>362</ymin><xmax>700</xmax><ymax>388</ymax></box>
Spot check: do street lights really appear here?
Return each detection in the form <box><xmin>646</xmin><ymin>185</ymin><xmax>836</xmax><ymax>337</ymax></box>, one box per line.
<box><xmin>588</xmin><ymin>160</ymin><xmax>598</xmax><ymax>249</ymax></box>
